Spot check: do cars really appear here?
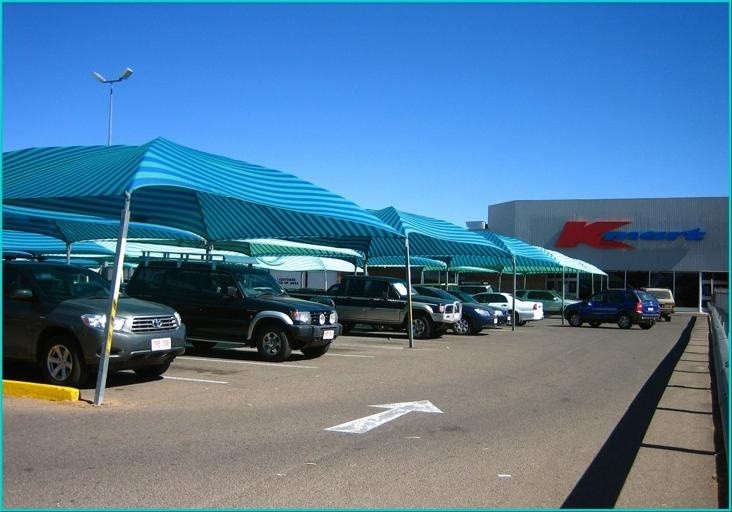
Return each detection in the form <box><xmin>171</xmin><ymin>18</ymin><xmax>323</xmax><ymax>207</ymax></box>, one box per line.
<box><xmin>562</xmin><ymin>288</ymin><xmax>661</xmax><ymax>333</ymax></box>
<box><xmin>317</xmin><ymin>283</ymin><xmax>340</xmax><ymax>292</ymax></box>
<box><xmin>407</xmin><ymin>280</ymin><xmax>585</xmax><ymax>338</ymax></box>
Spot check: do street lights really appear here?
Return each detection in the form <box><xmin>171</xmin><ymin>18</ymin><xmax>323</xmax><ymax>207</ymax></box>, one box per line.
<box><xmin>91</xmin><ymin>65</ymin><xmax>136</xmax><ymax>146</ymax></box>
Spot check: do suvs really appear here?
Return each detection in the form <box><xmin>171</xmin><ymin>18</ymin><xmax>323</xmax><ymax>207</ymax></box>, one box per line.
<box><xmin>121</xmin><ymin>245</ymin><xmax>344</xmax><ymax>366</ymax></box>
<box><xmin>2</xmin><ymin>250</ymin><xmax>189</xmax><ymax>392</ymax></box>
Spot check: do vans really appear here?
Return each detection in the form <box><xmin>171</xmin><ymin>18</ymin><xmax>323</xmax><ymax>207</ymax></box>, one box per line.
<box><xmin>637</xmin><ymin>285</ymin><xmax>677</xmax><ymax>324</ymax></box>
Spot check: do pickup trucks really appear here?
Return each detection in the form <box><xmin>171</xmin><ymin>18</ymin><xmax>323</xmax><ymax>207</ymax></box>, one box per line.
<box><xmin>282</xmin><ymin>272</ymin><xmax>465</xmax><ymax>339</ymax></box>
